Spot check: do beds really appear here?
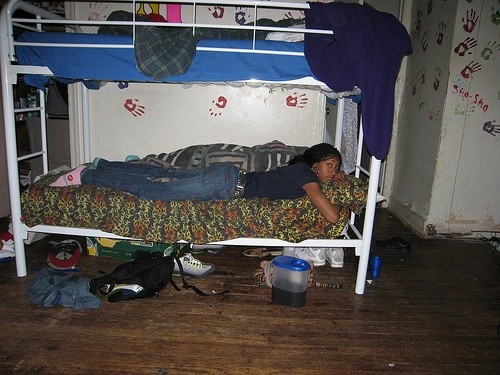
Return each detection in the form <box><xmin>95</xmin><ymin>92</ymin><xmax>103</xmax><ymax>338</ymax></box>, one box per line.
<box><xmin>0</xmin><ymin>0</ymin><xmax>413</xmax><ymax>294</ymax></box>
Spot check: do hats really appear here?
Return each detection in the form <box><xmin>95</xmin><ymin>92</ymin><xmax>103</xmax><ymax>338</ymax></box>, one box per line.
<box><xmin>46</xmin><ymin>239</ymin><xmax>83</xmax><ymax>271</ymax></box>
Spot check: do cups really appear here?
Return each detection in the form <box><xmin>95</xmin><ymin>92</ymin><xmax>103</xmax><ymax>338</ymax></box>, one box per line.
<box><xmin>370</xmin><ymin>256</ymin><xmax>383</xmax><ymax>278</ymax></box>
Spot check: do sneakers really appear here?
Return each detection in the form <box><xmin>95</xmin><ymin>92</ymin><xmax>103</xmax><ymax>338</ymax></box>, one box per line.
<box><xmin>325</xmin><ymin>248</ymin><xmax>344</xmax><ymax>268</ymax></box>
<box><xmin>294</xmin><ymin>247</ymin><xmax>326</xmax><ymax>267</ymax></box>
<box><xmin>173</xmin><ymin>253</ymin><xmax>216</xmax><ymax>278</ymax></box>
<box><xmin>376</xmin><ymin>236</ymin><xmax>412</xmax><ymax>253</ymax></box>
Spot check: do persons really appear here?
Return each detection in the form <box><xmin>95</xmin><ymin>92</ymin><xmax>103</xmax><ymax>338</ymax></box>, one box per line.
<box><xmin>52</xmin><ymin>143</ymin><xmax>341</xmax><ymax>223</ymax></box>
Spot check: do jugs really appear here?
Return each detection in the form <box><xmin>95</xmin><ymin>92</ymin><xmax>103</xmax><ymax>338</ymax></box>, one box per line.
<box><xmin>264</xmin><ymin>256</ymin><xmax>311</xmax><ymax>308</ymax></box>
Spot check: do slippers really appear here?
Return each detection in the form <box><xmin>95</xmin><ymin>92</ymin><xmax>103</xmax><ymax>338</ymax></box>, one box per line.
<box><xmin>243</xmin><ymin>247</ymin><xmax>283</xmax><ymax>258</ymax></box>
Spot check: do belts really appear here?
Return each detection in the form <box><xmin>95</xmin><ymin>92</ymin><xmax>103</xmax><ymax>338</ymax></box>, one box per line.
<box><xmin>233</xmin><ymin>169</ymin><xmax>247</xmax><ymax>199</ymax></box>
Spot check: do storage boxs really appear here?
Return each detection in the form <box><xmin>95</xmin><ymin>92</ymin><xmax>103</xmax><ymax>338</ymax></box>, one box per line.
<box><xmin>85</xmin><ymin>237</ymin><xmax>190</xmax><ymax>262</ymax></box>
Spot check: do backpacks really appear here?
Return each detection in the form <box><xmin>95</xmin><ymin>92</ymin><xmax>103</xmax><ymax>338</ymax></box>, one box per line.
<box><xmin>88</xmin><ymin>241</ymin><xmax>231</xmax><ymax>302</ymax></box>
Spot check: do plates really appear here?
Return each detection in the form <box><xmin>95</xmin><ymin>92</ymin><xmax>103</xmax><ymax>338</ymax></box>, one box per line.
<box><xmin>243</xmin><ymin>249</ymin><xmax>269</xmax><ymax>256</ymax></box>
